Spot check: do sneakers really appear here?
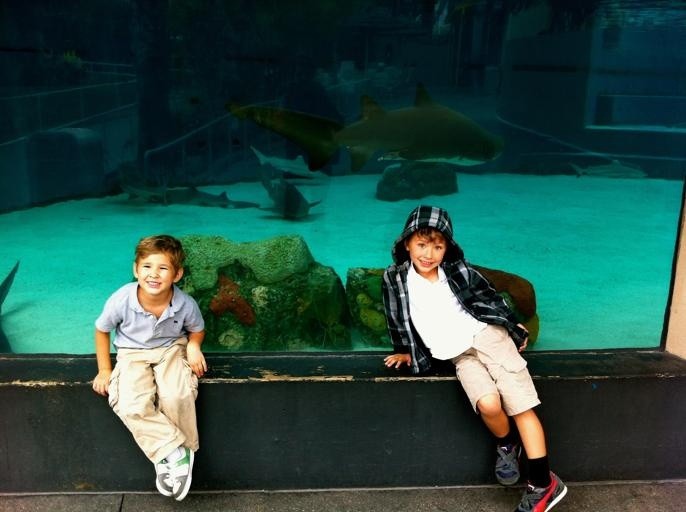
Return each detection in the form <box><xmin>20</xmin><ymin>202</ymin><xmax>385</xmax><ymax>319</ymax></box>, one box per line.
<box><xmin>495</xmin><ymin>443</ymin><xmax>521</xmax><ymax>485</ymax></box>
<box><xmin>515</xmin><ymin>471</ymin><xmax>568</xmax><ymax>512</ymax></box>
<box><xmin>155</xmin><ymin>459</ymin><xmax>173</xmax><ymax>496</ymax></box>
<box><xmin>174</xmin><ymin>447</ymin><xmax>195</xmax><ymax>502</ymax></box>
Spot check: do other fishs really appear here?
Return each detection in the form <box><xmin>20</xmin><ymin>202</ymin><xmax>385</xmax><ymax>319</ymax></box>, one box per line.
<box><xmin>568</xmin><ymin>161</ymin><xmax>648</xmax><ymax>179</ymax></box>
<box><xmin>42</xmin><ymin>50</ymin><xmax>136</xmax><ymax>74</ymax></box>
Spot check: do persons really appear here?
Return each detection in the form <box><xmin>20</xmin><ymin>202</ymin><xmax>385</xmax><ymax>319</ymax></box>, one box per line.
<box><xmin>385</xmin><ymin>205</ymin><xmax>567</xmax><ymax>512</ymax></box>
<box><xmin>92</xmin><ymin>235</ymin><xmax>209</xmax><ymax>503</ymax></box>
